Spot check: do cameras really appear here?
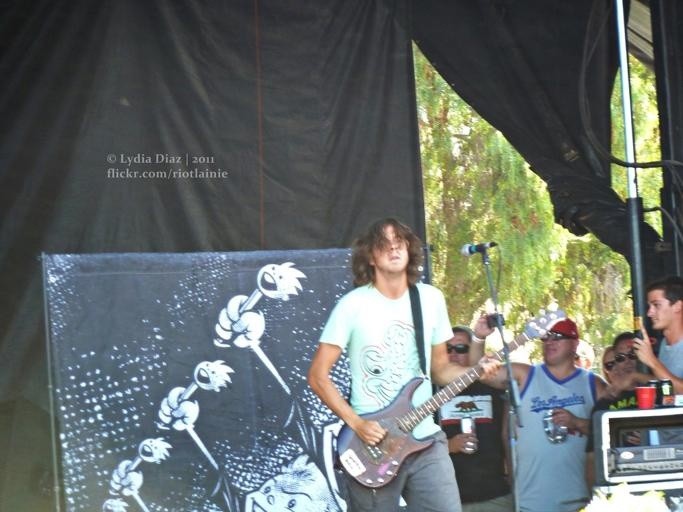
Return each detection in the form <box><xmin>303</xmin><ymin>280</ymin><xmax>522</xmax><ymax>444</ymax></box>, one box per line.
<box><xmin>488</xmin><ymin>313</ymin><xmax>506</xmax><ymax>328</ymax></box>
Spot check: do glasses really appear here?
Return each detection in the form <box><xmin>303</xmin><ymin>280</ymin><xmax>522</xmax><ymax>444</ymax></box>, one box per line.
<box><xmin>614</xmin><ymin>351</ymin><xmax>639</xmax><ymax>362</ymax></box>
<box><xmin>602</xmin><ymin>360</ymin><xmax>614</xmax><ymax>372</ymax></box>
<box><xmin>447</xmin><ymin>342</ymin><xmax>469</xmax><ymax>355</ymax></box>
<box><xmin>540</xmin><ymin>333</ymin><xmax>577</xmax><ymax>342</ymax></box>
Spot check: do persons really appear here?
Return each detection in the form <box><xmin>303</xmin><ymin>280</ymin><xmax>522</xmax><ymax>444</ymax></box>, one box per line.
<box><xmin>306</xmin><ymin>218</ymin><xmax>501</xmax><ymax>511</ymax></box>
<box><xmin>613</xmin><ymin>273</ymin><xmax>683</xmax><ymax>396</ymax></box>
<box><xmin>600</xmin><ymin>345</ymin><xmax>623</xmax><ymax>384</ymax></box>
<box><xmin>465</xmin><ymin>307</ymin><xmax>610</xmax><ymax>512</ymax></box>
<box><xmin>582</xmin><ymin>331</ymin><xmax>650</xmax><ymax>498</ymax></box>
<box><xmin>430</xmin><ymin>323</ymin><xmax>512</xmax><ymax>512</ymax></box>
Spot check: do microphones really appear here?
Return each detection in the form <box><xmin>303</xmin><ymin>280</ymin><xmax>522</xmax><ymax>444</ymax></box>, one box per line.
<box><xmin>460</xmin><ymin>243</ymin><xmax>497</xmax><ymax>257</ymax></box>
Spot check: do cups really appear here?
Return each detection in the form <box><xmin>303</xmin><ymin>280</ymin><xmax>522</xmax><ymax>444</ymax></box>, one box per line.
<box><xmin>634</xmin><ymin>386</ymin><xmax>656</xmax><ymax>409</ymax></box>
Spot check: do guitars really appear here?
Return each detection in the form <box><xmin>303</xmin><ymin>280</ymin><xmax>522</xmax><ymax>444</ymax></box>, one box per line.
<box><xmin>337</xmin><ymin>302</ymin><xmax>568</xmax><ymax>488</ymax></box>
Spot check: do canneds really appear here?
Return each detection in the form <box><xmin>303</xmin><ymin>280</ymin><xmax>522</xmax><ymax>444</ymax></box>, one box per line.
<box><xmin>659</xmin><ymin>378</ymin><xmax>674</xmax><ymax>406</ymax></box>
<box><xmin>648</xmin><ymin>378</ymin><xmax>661</xmax><ymax>404</ymax></box>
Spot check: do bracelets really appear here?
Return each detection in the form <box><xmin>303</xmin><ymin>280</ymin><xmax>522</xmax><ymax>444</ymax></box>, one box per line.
<box><xmin>470</xmin><ymin>335</ymin><xmax>484</xmax><ymax>344</ymax></box>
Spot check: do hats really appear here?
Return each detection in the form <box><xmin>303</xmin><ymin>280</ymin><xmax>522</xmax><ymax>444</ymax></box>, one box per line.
<box><xmin>451</xmin><ymin>324</ymin><xmax>474</xmax><ymax>341</ymax></box>
<box><xmin>550</xmin><ymin>317</ymin><xmax>579</xmax><ymax>340</ymax></box>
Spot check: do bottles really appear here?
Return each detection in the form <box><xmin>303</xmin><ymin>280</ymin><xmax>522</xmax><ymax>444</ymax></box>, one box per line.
<box><xmin>461</xmin><ymin>414</ymin><xmax>478</xmax><ymax>455</ymax></box>
